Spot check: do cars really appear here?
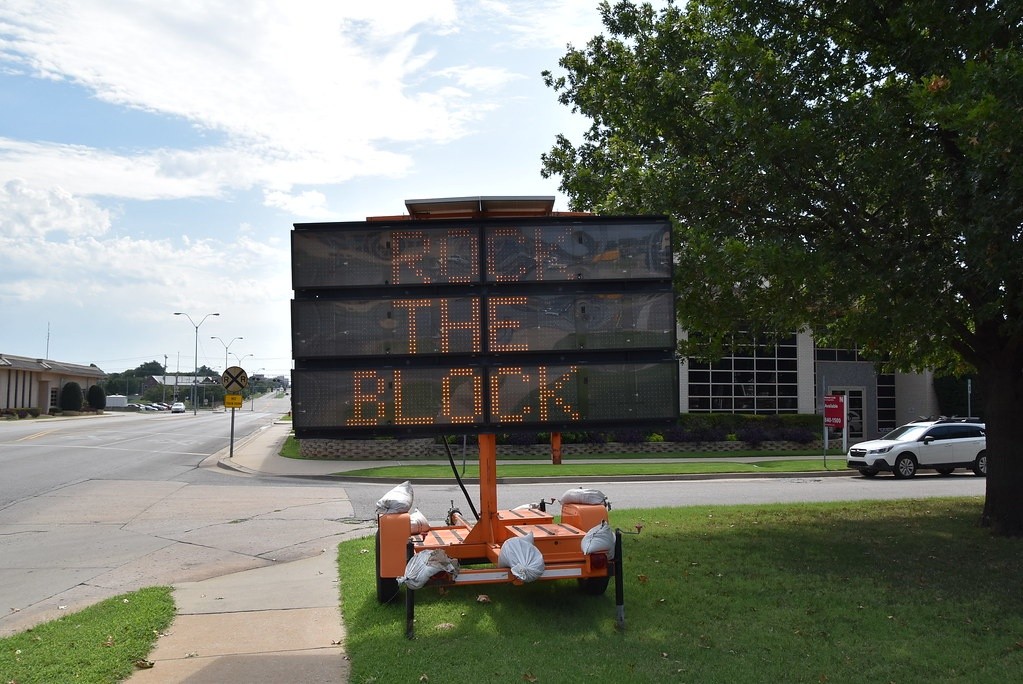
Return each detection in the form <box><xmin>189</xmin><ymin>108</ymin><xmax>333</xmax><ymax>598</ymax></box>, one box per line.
<box><xmin>127</xmin><ymin>402</ymin><xmax>173</xmax><ymax>411</ymax></box>
<box><xmin>171</xmin><ymin>402</ymin><xmax>186</xmax><ymax>413</ymax></box>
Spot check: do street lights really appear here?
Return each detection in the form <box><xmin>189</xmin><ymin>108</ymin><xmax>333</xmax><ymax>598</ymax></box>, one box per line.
<box><xmin>247</xmin><ymin>368</ymin><xmax>266</xmax><ymax>410</ymax></box>
<box><xmin>211</xmin><ymin>337</ymin><xmax>245</xmax><ymax>369</ymax></box>
<box><xmin>172</xmin><ymin>312</ymin><xmax>220</xmax><ymax>415</ymax></box>
<box><xmin>228</xmin><ymin>352</ymin><xmax>254</xmax><ymax>368</ymax></box>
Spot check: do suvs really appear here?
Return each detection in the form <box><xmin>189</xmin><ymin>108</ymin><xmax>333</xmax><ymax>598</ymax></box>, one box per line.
<box><xmin>846</xmin><ymin>415</ymin><xmax>992</xmax><ymax>479</ymax></box>
